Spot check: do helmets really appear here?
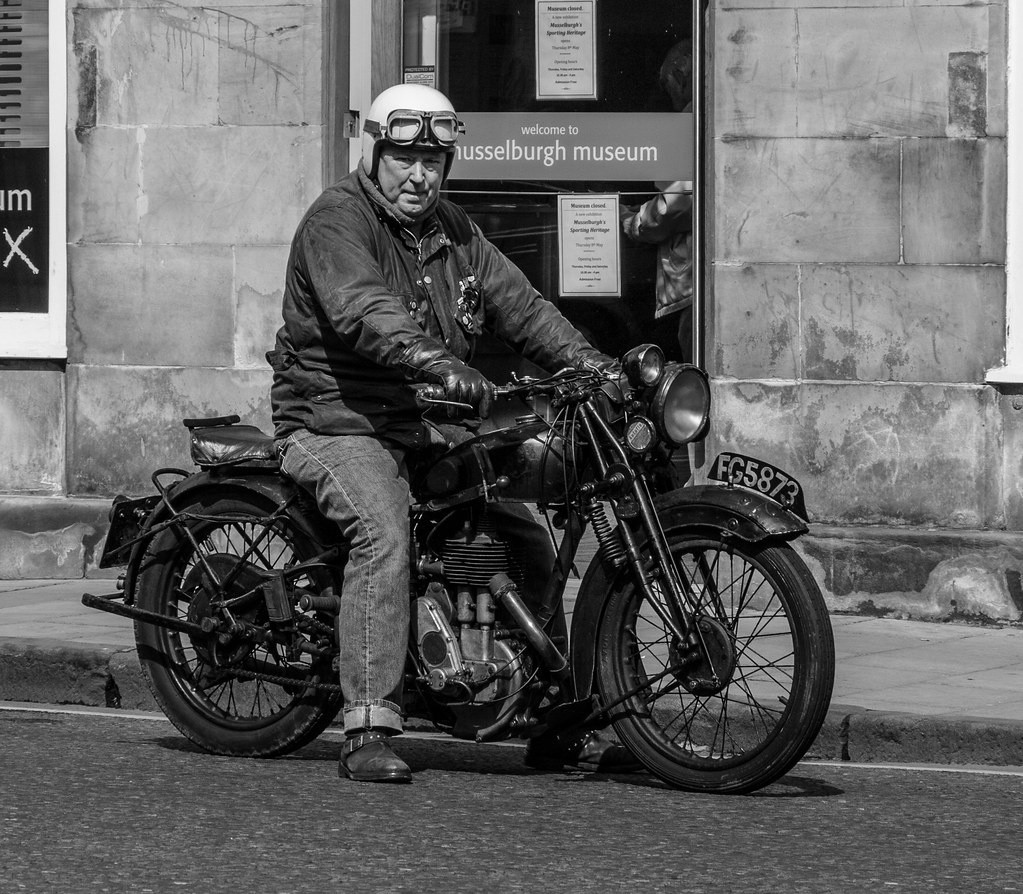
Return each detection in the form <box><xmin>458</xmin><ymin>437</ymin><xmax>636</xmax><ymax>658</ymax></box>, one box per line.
<box><xmin>362</xmin><ymin>84</ymin><xmax>458</xmax><ymax>181</ymax></box>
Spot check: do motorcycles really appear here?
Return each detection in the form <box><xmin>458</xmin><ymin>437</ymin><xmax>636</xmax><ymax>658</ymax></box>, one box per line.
<box><xmin>81</xmin><ymin>341</ymin><xmax>837</xmax><ymax>802</ymax></box>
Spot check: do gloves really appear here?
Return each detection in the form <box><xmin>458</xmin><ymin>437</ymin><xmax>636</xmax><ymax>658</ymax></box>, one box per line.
<box><xmin>580</xmin><ymin>353</ymin><xmax>630</xmax><ymax>380</ymax></box>
<box><xmin>398</xmin><ymin>337</ymin><xmax>493</xmax><ymax>419</ymax></box>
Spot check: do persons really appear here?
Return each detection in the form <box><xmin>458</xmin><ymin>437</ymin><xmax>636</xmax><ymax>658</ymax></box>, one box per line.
<box><xmin>264</xmin><ymin>83</ymin><xmax>641</xmax><ymax>785</ymax></box>
<box><xmin>620</xmin><ymin>41</ymin><xmax>694</xmax><ymax>366</ymax></box>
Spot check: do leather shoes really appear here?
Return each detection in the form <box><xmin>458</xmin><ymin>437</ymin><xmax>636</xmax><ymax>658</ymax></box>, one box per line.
<box><xmin>338</xmin><ymin>727</ymin><xmax>412</xmax><ymax>781</ymax></box>
<box><xmin>524</xmin><ymin>730</ymin><xmax>644</xmax><ymax>773</ymax></box>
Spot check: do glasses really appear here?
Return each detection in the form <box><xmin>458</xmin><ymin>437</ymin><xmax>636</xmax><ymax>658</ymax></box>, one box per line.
<box><xmin>386</xmin><ymin>107</ymin><xmax>458</xmax><ymax>143</ymax></box>
